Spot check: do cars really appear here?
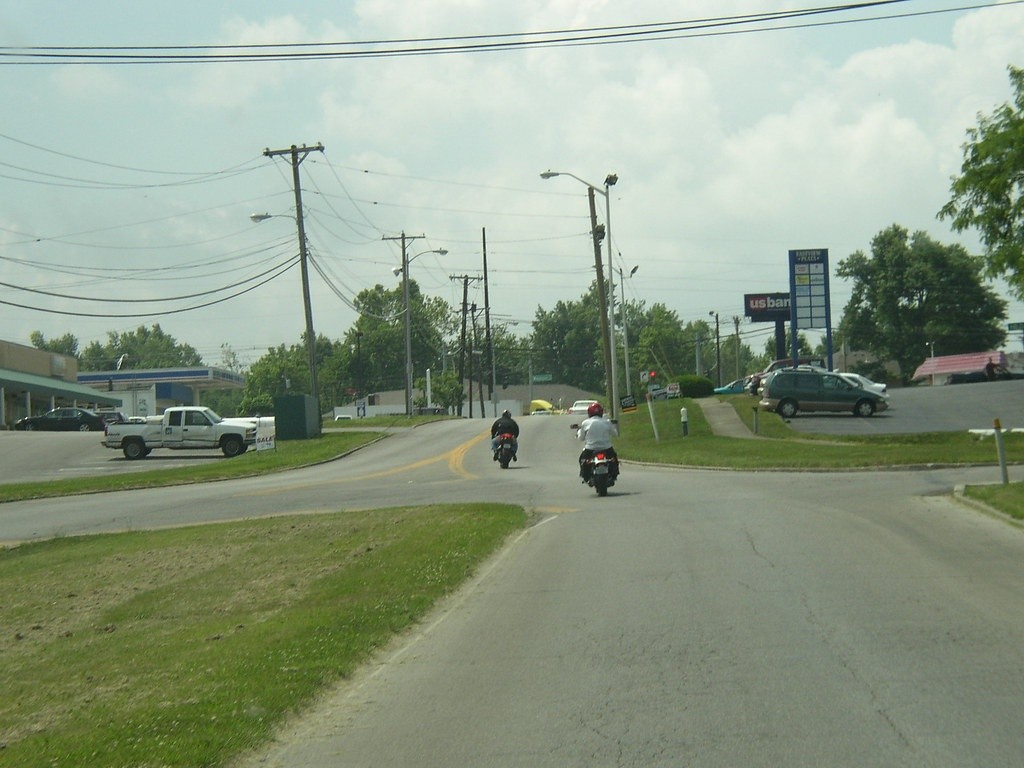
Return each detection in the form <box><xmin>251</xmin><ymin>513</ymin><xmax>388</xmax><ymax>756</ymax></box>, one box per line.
<box><xmin>92</xmin><ymin>411</ymin><xmax>166</xmax><ymax>425</ymax></box>
<box><xmin>756</xmin><ymin>364</ymin><xmax>890</xmax><ymax>400</ymax></box>
<box><xmin>568</xmin><ymin>400</ymin><xmax>597</xmax><ymax>414</ymax></box>
<box><xmin>335</xmin><ymin>414</ymin><xmax>353</xmax><ymax>422</ymax></box>
<box><xmin>713</xmin><ymin>379</ymin><xmax>744</xmax><ymax>395</ymax></box>
<box><xmin>14</xmin><ymin>407</ymin><xmax>107</xmax><ymax>432</ymax></box>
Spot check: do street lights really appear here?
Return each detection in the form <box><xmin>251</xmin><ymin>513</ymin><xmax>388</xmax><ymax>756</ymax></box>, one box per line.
<box><xmin>541</xmin><ymin>168</ymin><xmax>621</xmax><ymax>436</ymax></box>
<box><xmin>708</xmin><ymin>310</ymin><xmax>720</xmax><ymax>388</ymax></box>
<box><xmin>405</xmin><ymin>247</ymin><xmax>448</xmax><ymax>418</ymax></box>
<box><xmin>355</xmin><ymin>331</ymin><xmax>364</xmax><ymax>399</ymax></box>
<box><xmin>250</xmin><ymin>212</ymin><xmax>317</xmax><ymax>397</ymax></box>
<box><xmin>926</xmin><ymin>341</ymin><xmax>935</xmax><ymax>386</ymax></box>
<box><xmin>492</xmin><ymin>321</ymin><xmax>520</xmax><ymax>417</ymax></box>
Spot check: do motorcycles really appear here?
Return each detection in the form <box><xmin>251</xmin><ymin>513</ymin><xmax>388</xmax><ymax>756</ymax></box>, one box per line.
<box><xmin>492</xmin><ymin>432</ymin><xmax>515</xmax><ymax>469</ymax></box>
<box><xmin>570</xmin><ymin>420</ymin><xmax>619</xmax><ymax>498</ymax></box>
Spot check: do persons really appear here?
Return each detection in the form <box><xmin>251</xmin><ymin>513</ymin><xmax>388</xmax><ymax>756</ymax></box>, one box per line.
<box><xmin>986</xmin><ymin>356</ymin><xmax>997</xmax><ymax>381</ymax></box>
<box><xmin>577</xmin><ymin>403</ymin><xmax>619</xmax><ymax>484</ymax></box>
<box><xmin>491</xmin><ymin>409</ymin><xmax>519</xmax><ymax>462</ymax></box>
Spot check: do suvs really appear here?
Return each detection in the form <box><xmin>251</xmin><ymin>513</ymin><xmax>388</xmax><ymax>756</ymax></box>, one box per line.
<box><xmin>758</xmin><ymin>366</ymin><xmax>889</xmax><ymax>418</ymax></box>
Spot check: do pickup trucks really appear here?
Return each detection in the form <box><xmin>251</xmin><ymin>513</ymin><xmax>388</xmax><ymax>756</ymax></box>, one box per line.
<box><xmin>100</xmin><ymin>405</ymin><xmax>258</xmax><ymax>461</ymax></box>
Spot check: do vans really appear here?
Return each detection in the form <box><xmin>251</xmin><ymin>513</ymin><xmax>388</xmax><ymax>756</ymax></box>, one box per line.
<box><xmin>748</xmin><ymin>356</ymin><xmax>826</xmax><ymax>396</ymax></box>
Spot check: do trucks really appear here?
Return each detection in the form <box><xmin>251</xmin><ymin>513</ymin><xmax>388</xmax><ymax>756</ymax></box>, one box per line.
<box><xmin>448</xmin><ymin>399</ymin><xmax>552</xmax><ymax>418</ymax></box>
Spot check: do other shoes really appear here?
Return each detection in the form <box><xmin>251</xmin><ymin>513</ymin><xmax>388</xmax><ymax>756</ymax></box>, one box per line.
<box><xmin>513</xmin><ymin>455</ymin><xmax>517</xmax><ymax>461</ymax></box>
<box><xmin>494</xmin><ymin>454</ymin><xmax>498</xmax><ymax>460</ymax></box>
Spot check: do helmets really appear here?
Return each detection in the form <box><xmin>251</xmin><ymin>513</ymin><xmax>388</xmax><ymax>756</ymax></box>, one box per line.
<box><xmin>502</xmin><ymin>410</ymin><xmax>511</xmax><ymax>418</ymax></box>
<box><xmin>588</xmin><ymin>403</ymin><xmax>603</xmax><ymax>417</ymax></box>
